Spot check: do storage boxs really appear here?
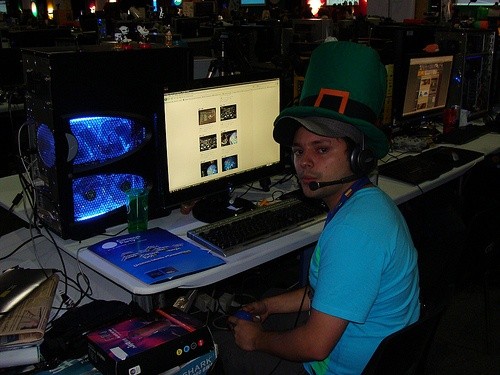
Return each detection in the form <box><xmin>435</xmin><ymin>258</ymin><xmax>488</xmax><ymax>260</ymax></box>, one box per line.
<box><xmin>86</xmin><ymin>304</ymin><xmax>215</xmax><ymax>375</ymax></box>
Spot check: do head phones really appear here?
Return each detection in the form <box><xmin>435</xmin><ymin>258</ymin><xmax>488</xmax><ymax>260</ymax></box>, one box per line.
<box><xmin>349</xmin><ymin>133</ymin><xmax>378</xmax><ymax>178</ymax></box>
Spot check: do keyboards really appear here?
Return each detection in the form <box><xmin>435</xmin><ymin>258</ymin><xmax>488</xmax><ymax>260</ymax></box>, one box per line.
<box><xmin>439</xmin><ymin>123</ymin><xmax>491</xmax><ymax>146</ymax></box>
<box><xmin>380</xmin><ymin>145</ymin><xmax>485</xmax><ymax>186</ymax></box>
<box><xmin>187</xmin><ymin>196</ymin><xmax>329</xmax><ymax>258</ymax></box>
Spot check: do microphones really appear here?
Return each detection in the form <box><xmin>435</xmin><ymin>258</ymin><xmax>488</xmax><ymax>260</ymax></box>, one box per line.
<box><xmin>309</xmin><ymin>176</ymin><xmax>358</xmax><ymax>192</ymax></box>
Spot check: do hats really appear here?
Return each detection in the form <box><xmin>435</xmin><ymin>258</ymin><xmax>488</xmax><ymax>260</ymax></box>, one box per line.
<box><xmin>274</xmin><ymin>40</ymin><xmax>392</xmax><ymax>159</ymax></box>
<box><xmin>273</xmin><ymin>114</ymin><xmax>366</xmax><ymax>149</ymax></box>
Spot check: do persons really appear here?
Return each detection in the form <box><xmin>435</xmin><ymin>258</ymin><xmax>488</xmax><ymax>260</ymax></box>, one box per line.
<box><xmin>220</xmin><ymin>105</ymin><xmax>421</xmax><ymax>375</ymax></box>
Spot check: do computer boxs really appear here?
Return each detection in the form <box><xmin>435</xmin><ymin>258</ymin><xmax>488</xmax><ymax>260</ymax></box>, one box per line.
<box><xmin>370</xmin><ymin>22</ymin><xmax>496</xmax><ymax>123</ymax></box>
<box><xmin>21</xmin><ymin>42</ymin><xmax>195</xmax><ymax>242</ymax></box>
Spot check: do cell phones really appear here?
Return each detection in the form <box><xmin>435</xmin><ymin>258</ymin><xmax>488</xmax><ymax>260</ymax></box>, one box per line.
<box><xmin>226</xmin><ymin>305</ymin><xmax>261</xmax><ymax>323</ymax></box>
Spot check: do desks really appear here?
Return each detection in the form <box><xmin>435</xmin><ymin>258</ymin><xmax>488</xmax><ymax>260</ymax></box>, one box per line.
<box><xmin>0</xmin><ymin>15</ymin><xmax>500</xmax><ymax>375</ymax></box>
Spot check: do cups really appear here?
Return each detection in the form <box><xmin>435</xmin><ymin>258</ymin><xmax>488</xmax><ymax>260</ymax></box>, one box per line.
<box><xmin>125</xmin><ymin>188</ymin><xmax>150</xmax><ymax>233</ymax></box>
<box><xmin>442</xmin><ymin>108</ymin><xmax>456</xmax><ymax>133</ymax></box>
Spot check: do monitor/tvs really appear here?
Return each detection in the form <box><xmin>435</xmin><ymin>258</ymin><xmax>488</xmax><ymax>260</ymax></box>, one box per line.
<box><xmin>158</xmin><ymin>71</ymin><xmax>286</xmax><ymax>222</ymax></box>
<box><xmin>402</xmin><ymin>55</ymin><xmax>453</xmax><ymax>136</ymax></box>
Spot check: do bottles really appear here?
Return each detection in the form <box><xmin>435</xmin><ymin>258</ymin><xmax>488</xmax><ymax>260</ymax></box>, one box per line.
<box><xmin>165</xmin><ymin>30</ymin><xmax>173</xmax><ymax>47</ymax></box>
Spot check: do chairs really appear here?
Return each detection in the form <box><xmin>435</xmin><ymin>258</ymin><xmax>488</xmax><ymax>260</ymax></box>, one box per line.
<box><xmin>360</xmin><ymin>291</ymin><xmax>440</xmax><ymax>375</ymax></box>
